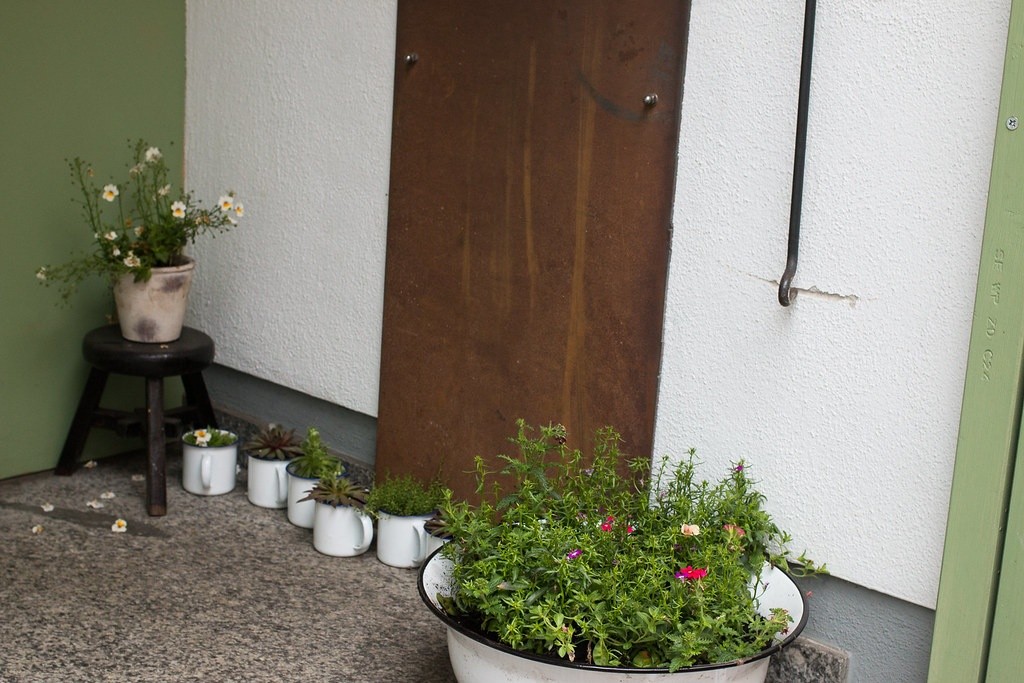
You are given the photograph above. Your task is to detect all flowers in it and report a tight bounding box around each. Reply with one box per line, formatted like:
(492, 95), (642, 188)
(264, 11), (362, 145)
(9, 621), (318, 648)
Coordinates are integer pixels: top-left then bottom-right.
(184, 426), (237, 446)
(35, 136), (245, 311)
(424, 420), (829, 666)
(33, 461), (145, 534)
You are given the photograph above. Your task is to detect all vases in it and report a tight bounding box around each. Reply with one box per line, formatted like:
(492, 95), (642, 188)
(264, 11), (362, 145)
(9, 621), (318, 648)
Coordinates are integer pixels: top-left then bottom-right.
(113, 254), (196, 342)
(182, 430), (238, 497)
(417, 519), (809, 683)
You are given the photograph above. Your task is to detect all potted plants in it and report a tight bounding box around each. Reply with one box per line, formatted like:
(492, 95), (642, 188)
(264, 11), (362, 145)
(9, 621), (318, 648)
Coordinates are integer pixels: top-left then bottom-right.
(285, 426), (350, 528)
(243, 424), (305, 509)
(296, 478), (374, 557)
(359, 470), (451, 569)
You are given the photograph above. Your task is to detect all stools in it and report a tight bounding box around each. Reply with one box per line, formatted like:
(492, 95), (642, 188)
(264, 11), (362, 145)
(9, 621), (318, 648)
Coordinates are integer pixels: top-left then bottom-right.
(55, 322), (215, 517)
